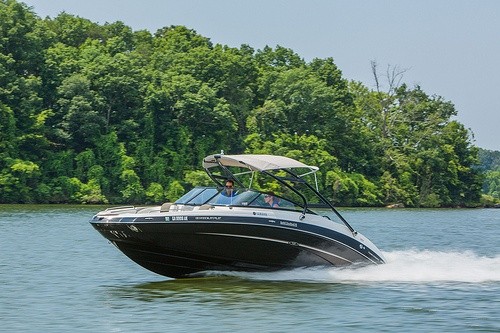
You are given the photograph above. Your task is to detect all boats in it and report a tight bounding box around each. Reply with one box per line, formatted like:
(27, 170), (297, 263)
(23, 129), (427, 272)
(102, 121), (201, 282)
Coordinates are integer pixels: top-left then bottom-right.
(89, 149), (387, 279)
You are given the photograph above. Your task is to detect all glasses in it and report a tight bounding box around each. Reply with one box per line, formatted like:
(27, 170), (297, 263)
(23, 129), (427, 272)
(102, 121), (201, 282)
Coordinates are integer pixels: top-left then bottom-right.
(225, 186), (233, 188)
(264, 196), (271, 198)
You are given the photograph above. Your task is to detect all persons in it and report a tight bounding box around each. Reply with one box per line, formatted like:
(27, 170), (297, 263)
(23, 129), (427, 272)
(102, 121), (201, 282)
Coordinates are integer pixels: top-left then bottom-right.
(213, 179), (241, 206)
(264, 191), (282, 209)
